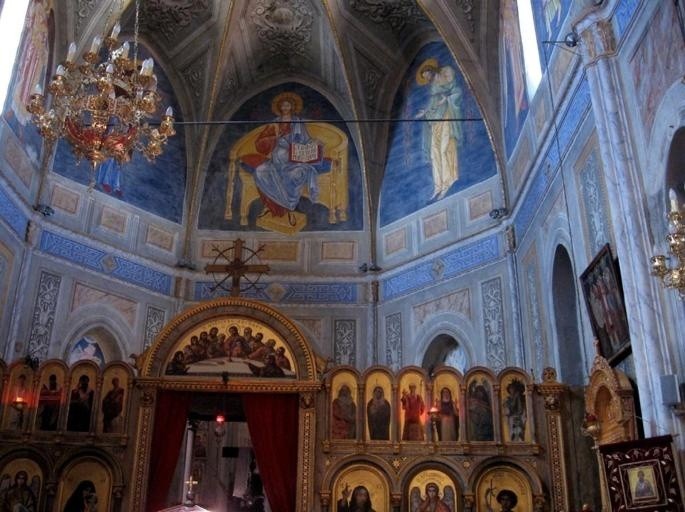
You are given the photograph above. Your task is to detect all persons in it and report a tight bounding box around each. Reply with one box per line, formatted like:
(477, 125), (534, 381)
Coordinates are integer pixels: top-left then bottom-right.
(5, 374), (29, 432)
(6, 0), (50, 141)
(66, 374), (93, 432)
(484, 484), (518, 512)
(502, 381), (528, 442)
(96, 117), (133, 199)
(434, 386), (459, 441)
(332, 383), (356, 438)
(415, 58), (465, 202)
(35, 374), (63, 433)
(61, 479), (98, 511)
(101, 376), (124, 433)
(336, 480), (375, 511)
(253, 91), (324, 227)
(468, 384), (494, 441)
(401, 382), (426, 441)
(0, 468), (37, 512)
(167, 324), (292, 378)
(202, 433), (208, 457)
(413, 480), (451, 511)
(634, 470), (653, 497)
(195, 434), (206, 457)
(582, 258), (632, 362)
(367, 384), (391, 440)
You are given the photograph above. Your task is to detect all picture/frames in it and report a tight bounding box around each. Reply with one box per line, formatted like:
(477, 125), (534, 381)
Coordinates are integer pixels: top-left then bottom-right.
(579, 243), (632, 367)
(595, 433), (685, 512)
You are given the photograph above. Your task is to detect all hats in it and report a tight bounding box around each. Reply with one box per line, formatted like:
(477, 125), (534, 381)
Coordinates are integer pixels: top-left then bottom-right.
(416, 58), (438, 86)
(272, 92), (303, 115)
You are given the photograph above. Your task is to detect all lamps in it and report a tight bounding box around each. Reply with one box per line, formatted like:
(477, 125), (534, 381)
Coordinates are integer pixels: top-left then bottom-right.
(26, 1), (177, 171)
(11, 397), (27, 430)
(214, 392), (226, 438)
(649, 188), (685, 309)
(427, 406), (442, 443)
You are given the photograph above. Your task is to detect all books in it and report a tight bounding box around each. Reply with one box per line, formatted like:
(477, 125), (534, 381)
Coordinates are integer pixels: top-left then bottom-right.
(288, 141), (322, 163)
